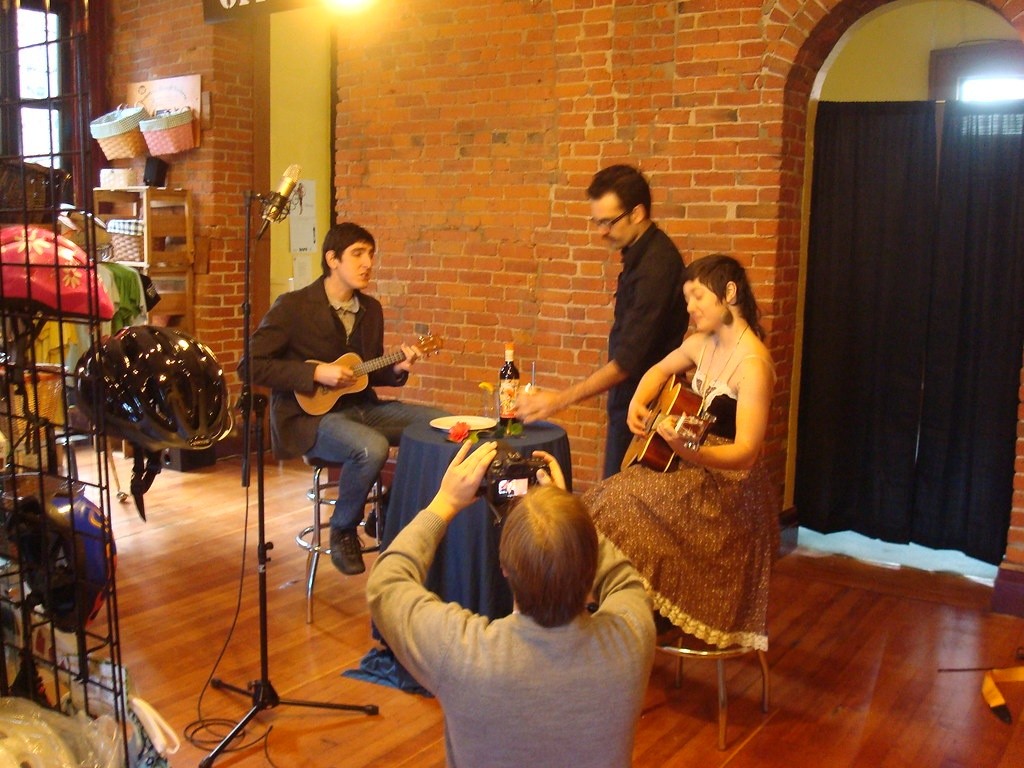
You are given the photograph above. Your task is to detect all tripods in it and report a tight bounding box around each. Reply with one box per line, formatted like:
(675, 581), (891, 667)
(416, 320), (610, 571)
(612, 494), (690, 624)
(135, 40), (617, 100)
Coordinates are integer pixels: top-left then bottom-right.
(199, 188), (376, 768)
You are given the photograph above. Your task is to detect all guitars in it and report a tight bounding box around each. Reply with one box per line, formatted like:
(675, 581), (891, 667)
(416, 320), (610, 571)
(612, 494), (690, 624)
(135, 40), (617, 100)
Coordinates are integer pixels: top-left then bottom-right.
(293, 330), (444, 416)
(619, 373), (717, 472)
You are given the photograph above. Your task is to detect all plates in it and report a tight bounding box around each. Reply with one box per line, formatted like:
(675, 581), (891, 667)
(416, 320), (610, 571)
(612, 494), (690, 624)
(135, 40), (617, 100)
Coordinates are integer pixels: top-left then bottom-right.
(429, 415), (498, 432)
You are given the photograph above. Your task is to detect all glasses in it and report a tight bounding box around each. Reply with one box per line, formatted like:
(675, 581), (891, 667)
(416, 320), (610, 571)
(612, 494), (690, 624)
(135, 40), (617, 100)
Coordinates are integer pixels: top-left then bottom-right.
(590, 203), (638, 234)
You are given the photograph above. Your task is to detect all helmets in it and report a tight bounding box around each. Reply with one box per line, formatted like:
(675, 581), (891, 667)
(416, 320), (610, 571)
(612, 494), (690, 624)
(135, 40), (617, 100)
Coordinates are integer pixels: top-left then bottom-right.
(0, 224), (115, 321)
(6, 493), (119, 634)
(73, 326), (234, 449)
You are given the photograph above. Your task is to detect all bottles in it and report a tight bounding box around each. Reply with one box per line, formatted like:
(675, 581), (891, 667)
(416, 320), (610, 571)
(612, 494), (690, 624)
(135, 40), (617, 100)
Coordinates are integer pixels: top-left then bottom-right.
(499, 350), (520, 426)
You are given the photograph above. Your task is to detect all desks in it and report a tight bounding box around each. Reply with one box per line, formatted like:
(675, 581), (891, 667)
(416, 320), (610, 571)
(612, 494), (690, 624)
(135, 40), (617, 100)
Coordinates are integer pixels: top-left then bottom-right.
(392, 420), (571, 617)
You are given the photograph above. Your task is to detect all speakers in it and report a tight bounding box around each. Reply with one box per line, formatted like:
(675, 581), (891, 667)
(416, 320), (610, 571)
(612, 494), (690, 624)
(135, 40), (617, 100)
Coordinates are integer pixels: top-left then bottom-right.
(143, 157), (168, 187)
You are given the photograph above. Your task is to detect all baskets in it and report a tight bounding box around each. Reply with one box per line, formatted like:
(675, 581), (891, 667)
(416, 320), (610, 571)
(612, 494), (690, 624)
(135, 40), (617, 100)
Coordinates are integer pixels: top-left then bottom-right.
(107, 220), (165, 262)
(0, 159), (71, 224)
(90, 107), (151, 161)
(139, 106), (195, 157)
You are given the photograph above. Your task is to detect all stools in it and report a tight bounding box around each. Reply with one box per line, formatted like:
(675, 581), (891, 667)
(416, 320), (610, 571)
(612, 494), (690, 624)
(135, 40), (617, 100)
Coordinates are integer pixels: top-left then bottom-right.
(295, 455), (383, 623)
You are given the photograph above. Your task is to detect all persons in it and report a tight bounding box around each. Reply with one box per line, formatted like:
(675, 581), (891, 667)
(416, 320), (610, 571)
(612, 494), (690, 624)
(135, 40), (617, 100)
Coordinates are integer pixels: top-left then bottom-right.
(235, 222), (452, 575)
(367, 441), (655, 767)
(516, 163), (690, 481)
(580, 254), (778, 651)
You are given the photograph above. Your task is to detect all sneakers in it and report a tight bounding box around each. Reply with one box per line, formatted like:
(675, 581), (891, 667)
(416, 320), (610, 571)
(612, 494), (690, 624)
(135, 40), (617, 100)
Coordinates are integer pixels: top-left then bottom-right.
(365, 513), (383, 540)
(329, 526), (365, 575)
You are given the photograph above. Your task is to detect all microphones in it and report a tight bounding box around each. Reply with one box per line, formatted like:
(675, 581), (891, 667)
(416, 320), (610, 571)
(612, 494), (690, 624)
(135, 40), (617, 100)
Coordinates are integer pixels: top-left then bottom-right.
(255, 164), (303, 241)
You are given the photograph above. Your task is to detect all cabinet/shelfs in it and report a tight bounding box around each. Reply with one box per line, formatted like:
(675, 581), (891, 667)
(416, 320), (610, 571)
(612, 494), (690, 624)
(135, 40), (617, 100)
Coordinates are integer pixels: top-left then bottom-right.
(91, 187), (196, 458)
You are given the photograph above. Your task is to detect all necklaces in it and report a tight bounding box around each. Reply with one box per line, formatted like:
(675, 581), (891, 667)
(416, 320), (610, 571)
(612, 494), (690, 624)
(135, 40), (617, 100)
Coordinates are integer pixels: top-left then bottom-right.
(700, 325), (749, 393)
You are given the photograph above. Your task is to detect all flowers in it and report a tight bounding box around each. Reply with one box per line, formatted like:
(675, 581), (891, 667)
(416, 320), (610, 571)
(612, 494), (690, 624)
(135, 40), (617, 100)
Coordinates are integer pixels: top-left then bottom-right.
(447, 422), (471, 443)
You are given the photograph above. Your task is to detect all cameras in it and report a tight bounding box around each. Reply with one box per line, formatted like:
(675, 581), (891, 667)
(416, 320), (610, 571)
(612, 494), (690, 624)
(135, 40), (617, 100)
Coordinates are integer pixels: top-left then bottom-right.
(476, 438), (550, 506)
